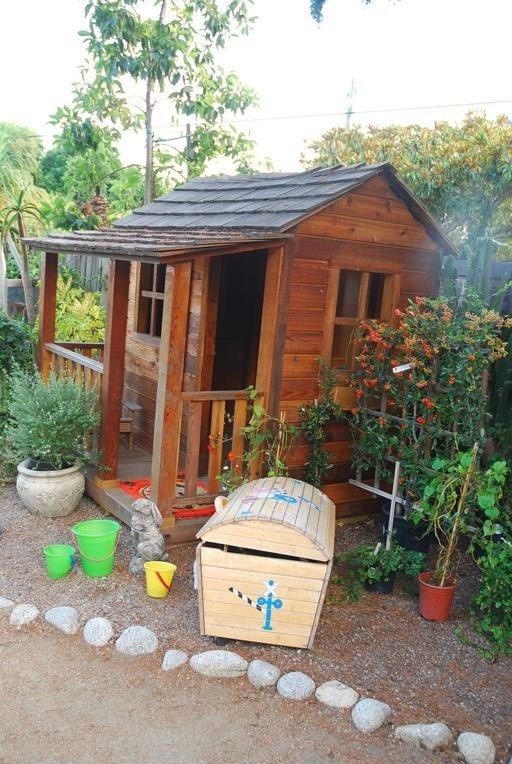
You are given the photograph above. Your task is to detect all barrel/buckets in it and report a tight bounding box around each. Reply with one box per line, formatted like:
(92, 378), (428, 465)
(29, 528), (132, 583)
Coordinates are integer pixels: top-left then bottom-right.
(418, 572), (458, 621)
(144, 561), (177, 598)
(44, 544), (74, 579)
(71, 520), (122, 576)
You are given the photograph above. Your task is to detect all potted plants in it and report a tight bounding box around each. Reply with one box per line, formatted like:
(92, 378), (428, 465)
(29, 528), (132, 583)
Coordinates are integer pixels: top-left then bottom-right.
(328, 434), (503, 623)
(2, 352), (112, 519)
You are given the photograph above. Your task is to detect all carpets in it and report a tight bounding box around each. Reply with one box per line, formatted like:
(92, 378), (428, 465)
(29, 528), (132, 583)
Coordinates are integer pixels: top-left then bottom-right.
(119, 471), (221, 518)
